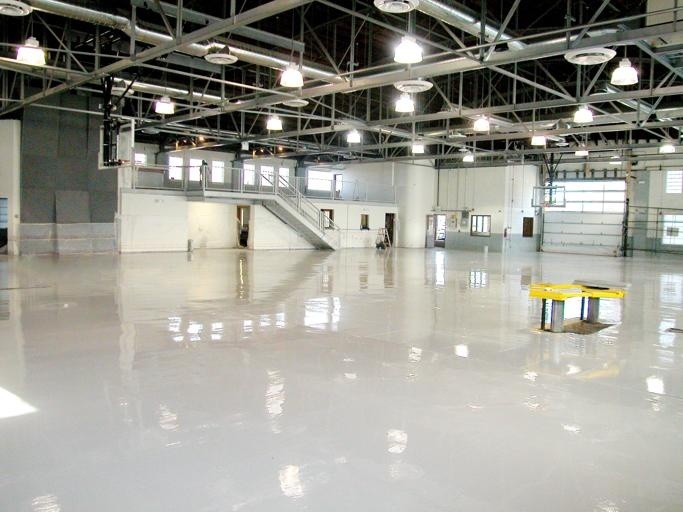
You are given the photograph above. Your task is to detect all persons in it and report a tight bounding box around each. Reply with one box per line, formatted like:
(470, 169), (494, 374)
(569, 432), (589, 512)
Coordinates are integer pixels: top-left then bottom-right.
(198, 159), (211, 190)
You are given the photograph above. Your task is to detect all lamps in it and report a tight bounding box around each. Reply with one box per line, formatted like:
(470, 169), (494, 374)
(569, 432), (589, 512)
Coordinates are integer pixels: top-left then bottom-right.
(574, 136), (589, 156)
(531, 109), (546, 146)
(16, 12), (46, 66)
(393, 12), (424, 64)
(412, 122), (423, 154)
(155, 59), (175, 114)
(462, 134), (475, 162)
(394, 92), (414, 113)
(346, 92), (361, 145)
(279, 9), (304, 88)
(572, 66), (594, 123)
(265, 105), (283, 130)
(659, 127), (675, 155)
(473, 69), (490, 131)
(610, 46), (639, 86)
